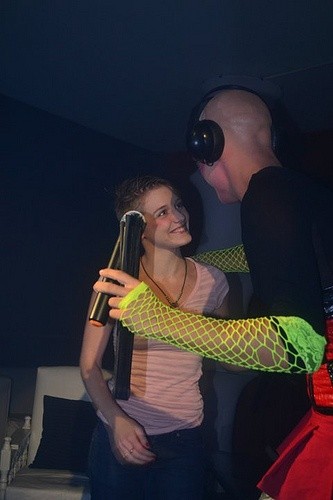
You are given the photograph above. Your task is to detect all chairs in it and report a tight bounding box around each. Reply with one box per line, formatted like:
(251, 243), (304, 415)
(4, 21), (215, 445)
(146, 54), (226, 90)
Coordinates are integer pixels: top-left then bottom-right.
(0, 367), (114, 500)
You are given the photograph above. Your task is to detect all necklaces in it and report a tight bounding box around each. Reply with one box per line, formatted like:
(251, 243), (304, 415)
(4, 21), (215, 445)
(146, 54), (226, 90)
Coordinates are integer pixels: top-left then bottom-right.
(139, 255), (188, 309)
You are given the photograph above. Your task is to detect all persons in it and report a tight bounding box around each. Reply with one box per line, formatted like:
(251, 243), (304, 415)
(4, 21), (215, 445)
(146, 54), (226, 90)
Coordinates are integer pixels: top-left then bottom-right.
(90, 88), (333, 500)
(78, 176), (249, 500)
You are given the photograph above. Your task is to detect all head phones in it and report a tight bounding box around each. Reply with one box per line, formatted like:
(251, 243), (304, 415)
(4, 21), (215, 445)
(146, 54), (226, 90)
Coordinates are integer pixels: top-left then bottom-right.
(185, 84), (283, 166)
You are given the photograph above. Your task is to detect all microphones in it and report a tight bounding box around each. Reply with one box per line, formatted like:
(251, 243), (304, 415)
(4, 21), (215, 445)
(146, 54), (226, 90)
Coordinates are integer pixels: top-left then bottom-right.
(88, 210), (146, 327)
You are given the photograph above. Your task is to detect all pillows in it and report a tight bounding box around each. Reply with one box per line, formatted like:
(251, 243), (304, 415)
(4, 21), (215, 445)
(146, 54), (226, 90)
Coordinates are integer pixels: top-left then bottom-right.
(29, 396), (97, 473)
